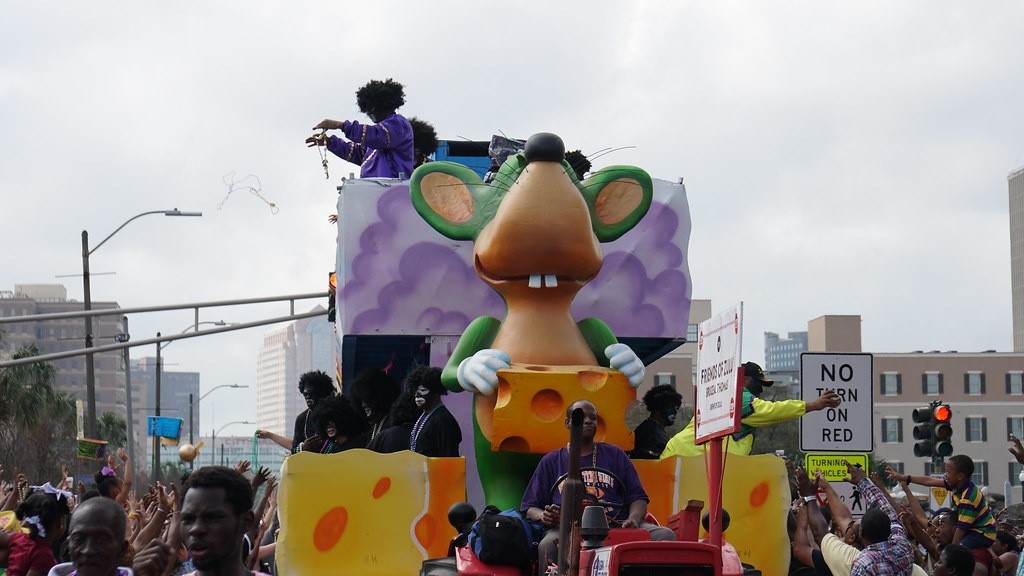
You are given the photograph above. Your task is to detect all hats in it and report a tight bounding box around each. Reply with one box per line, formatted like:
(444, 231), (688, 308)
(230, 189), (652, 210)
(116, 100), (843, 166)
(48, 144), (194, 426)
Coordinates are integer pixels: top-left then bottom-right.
(743, 362), (774, 387)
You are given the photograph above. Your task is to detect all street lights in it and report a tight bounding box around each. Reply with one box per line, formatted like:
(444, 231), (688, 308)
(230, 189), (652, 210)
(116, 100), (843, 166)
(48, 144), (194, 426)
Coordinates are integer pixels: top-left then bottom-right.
(1019, 470), (1024, 503)
(211, 420), (256, 467)
(153, 320), (242, 490)
(79, 207), (206, 473)
(187, 384), (250, 471)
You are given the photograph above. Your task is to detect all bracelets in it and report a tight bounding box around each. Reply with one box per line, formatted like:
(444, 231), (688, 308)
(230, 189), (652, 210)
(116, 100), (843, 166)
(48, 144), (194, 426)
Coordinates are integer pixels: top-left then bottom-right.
(297, 442), (304, 452)
(906, 475), (911, 486)
(803, 495), (817, 504)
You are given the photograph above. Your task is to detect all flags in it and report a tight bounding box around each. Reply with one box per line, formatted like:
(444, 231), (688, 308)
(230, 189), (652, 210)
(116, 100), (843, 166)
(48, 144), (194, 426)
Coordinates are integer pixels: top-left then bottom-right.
(77, 440), (108, 460)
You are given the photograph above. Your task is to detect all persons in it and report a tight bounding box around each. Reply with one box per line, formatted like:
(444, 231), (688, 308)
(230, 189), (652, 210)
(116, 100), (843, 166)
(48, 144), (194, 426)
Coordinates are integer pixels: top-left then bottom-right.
(291, 367), (463, 457)
(520, 400), (676, 576)
(787, 433), (1024, 576)
(0, 448), (280, 576)
(305, 79), (439, 224)
(255, 429), (292, 450)
(659, 362), (839, 460)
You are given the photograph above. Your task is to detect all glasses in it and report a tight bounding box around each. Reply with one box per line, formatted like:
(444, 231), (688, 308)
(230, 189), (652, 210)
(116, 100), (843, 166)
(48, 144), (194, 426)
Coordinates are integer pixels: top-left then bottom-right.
(848, 521), (856, 538)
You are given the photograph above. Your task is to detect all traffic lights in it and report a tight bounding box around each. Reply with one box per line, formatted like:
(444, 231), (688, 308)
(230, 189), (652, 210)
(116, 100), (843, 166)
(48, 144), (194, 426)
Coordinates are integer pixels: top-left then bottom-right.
(930, 405), (953, 457)
(327, 271), (336, 323)
(911, 406), (933, 458)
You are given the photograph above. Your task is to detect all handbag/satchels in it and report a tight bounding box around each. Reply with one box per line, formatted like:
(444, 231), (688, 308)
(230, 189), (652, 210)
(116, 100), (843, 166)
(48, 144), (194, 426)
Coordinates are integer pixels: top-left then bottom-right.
(467, 505), (534, 567)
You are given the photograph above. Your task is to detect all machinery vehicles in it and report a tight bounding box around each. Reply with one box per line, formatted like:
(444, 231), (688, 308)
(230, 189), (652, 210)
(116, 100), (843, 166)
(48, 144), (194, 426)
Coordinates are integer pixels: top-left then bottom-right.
(418, 407), (763, 576)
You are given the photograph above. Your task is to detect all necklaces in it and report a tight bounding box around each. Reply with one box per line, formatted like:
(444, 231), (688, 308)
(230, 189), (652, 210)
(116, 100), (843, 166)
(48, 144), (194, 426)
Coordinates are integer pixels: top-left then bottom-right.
(18, 481), (27, 502)
(314, 128), (329, 179)
(323, 442), (333, 454)
(372, 412), (389, 440)
(251, 430), (261, 475)
(422, 157), (427, 164)
(411, 400), (445, 452)
(567, 440), (596, 497)
(304, 410), (318, 439)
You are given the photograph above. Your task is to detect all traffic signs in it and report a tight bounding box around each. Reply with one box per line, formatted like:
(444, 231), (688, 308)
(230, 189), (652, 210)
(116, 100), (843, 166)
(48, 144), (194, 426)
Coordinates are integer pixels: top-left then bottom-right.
(804, 452), (869, 483)
